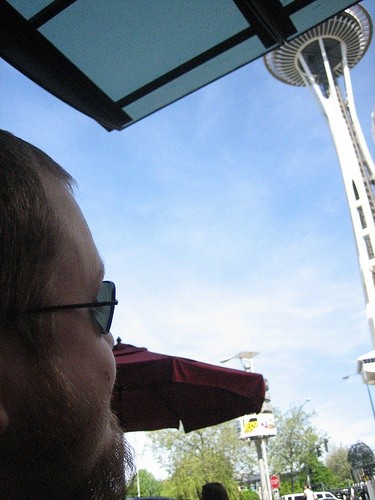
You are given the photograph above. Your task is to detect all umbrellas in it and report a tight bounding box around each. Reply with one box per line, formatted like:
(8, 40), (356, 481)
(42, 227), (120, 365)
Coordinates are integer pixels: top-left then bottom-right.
(111, 337), (265, 434)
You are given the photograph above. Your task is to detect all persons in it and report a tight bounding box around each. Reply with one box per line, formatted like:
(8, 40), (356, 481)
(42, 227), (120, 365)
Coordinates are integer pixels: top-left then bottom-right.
(200, 482), (229, 500)
(303, 484), (318, 500)
(1, 128), (136, 500)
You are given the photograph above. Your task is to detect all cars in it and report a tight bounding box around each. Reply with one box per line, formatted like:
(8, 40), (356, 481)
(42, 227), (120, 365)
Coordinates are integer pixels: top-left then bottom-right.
(281, 483), (369, 500)
